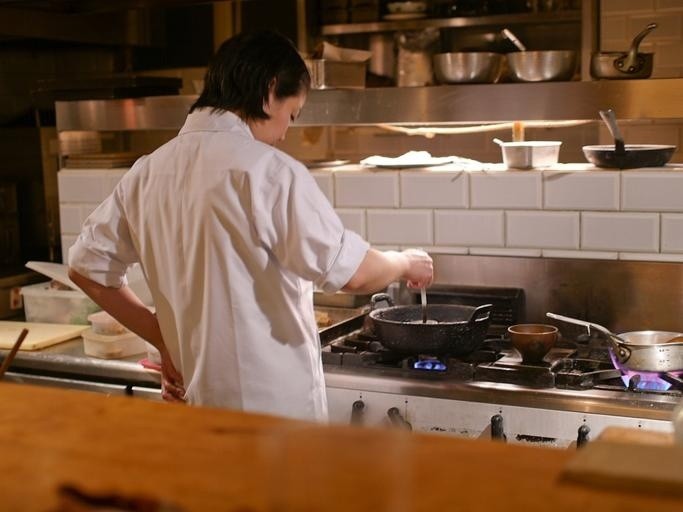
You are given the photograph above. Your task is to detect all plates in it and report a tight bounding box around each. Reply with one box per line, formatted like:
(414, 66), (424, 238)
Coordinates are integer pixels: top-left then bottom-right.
(360, 159), (454, 169)
(384, 11), (430, 21)
(307, 156), (349, 168)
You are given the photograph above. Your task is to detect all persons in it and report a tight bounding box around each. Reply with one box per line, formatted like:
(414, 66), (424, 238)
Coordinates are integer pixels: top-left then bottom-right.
(68, 28), (433, 426)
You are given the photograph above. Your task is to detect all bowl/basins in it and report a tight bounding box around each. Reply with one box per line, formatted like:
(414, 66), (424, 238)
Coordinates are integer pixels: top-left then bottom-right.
(430, 51), (506, 85)
(386, 1), (426, 12)
(506, 49), (579, 83)
(508, 323), (559, 364)
(500, 139), (564, 169)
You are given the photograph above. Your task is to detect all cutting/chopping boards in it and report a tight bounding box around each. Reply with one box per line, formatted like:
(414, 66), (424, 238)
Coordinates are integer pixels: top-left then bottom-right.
(0, 320), (92, 351)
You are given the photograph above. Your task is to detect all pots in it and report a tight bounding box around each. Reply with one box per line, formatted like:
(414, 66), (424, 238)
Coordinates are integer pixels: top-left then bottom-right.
(546, 312), (683, 373)
(368, 292), (494, 359)
(581, 108), (677, 170)
(589, 21), (658, 80)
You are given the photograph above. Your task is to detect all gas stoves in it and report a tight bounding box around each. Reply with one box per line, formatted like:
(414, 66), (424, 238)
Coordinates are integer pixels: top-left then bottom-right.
(315, 324), (683, 450)
